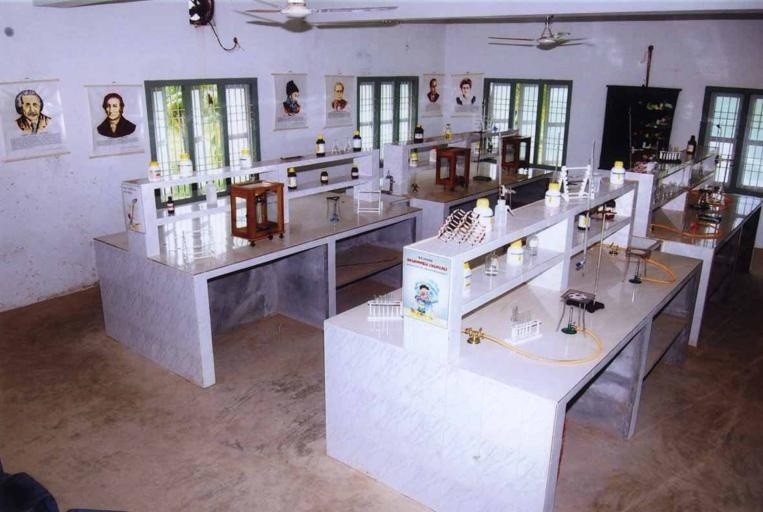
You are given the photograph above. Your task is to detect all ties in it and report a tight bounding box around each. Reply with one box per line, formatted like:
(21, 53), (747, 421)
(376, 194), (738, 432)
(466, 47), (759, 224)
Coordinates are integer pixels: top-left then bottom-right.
(32, 122), (37, 133)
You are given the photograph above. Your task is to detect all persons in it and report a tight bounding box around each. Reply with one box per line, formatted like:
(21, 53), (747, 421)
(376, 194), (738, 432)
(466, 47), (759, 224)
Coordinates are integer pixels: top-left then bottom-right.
(282, 80), (301, 117)
(425, 78), (440, 103)
(330, 82), (350, 113)
(11, 89), (53, 136)
(455, 78), (478, 106)
(95, 93), (135, 139)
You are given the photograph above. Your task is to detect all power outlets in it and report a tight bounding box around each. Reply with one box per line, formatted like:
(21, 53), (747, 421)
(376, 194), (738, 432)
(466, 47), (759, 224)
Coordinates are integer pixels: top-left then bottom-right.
(231, 33), (239, 45)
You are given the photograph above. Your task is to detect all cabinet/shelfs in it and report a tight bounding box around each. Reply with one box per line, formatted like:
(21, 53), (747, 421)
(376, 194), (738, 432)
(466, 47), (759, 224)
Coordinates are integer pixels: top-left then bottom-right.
(229, 180), (285, 245)
(403, 179), (639, 325)
(120, 147), (380, 237)
(614, 145), (720, 238)
(502, 136), (531, 172)
(384, 127), (517, 193)
(435, 147), (471, 191)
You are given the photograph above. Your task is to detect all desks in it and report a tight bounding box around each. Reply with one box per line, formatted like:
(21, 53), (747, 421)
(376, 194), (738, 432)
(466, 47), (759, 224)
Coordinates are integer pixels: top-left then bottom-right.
(324, 243), (704, 511)
(91, 192), (423, 389)
(631, 236), (662, 252)
(606, 190), (762, 348)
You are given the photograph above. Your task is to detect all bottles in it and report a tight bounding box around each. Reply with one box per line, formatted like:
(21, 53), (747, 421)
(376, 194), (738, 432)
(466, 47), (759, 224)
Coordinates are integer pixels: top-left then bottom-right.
(526, 235), (538, 257)
(686, 134), (696, 155)
(506, 240), (524, 269)
(288, 168), (297, 189)
(239, 147), (252, 167)
(413, 125), (424, 142)
(472, 197), (492, 236)
(350, 163), (359, 179)
(352, 130), (363, 152)
(442, 123), (452, 141)
(652, 181), (683, 206)
(370, 320), (390, 339)
(408, 149), (418, 169)
(544, 181), (561, 208)
(178, 153), (194, 178)
(494, 199), (508, 228)
(659, 151), (682, 163)
(315, 134), (325, 156)
(461, 263), (472, 299)
(509, 304), (535, 334)
(578, 212), (592, 231)
(320, 169), (328, 184)
(147, 160), (162, 182)
(429, 147), (437, 163)
(609, 161), (625, 187)
(370, 291), (397, 317)
(166, 196), (174, 214)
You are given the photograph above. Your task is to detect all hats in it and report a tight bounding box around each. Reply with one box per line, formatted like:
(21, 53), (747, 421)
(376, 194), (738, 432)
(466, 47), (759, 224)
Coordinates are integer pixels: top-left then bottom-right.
(286, 80), (299, 96)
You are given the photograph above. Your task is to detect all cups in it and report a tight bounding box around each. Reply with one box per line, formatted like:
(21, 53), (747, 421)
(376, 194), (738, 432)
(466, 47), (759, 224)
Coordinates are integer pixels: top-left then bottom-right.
(327, 196), (342, 225)
(485, 251), (497, 280)
(205, 184), (217, 208)
(588, 172), (602, 194)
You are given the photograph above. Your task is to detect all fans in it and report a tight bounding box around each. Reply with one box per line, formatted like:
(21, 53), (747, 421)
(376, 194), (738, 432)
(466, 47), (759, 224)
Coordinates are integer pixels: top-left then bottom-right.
(242, 0), (398, 19)
(488, 15), (588, 45)
